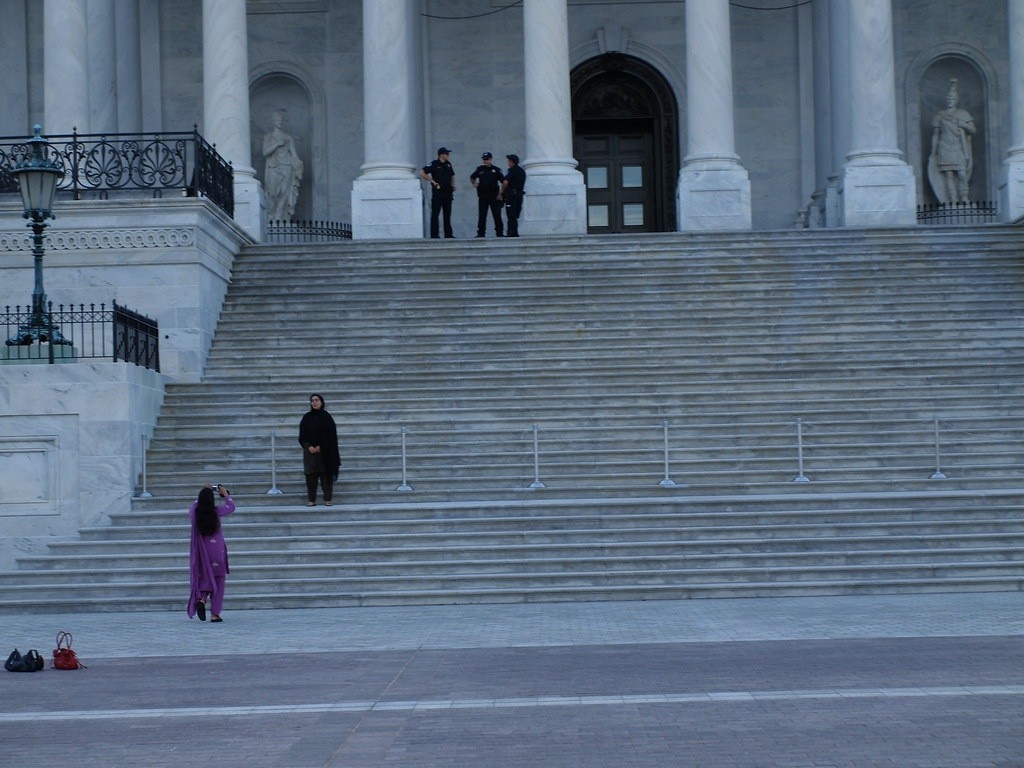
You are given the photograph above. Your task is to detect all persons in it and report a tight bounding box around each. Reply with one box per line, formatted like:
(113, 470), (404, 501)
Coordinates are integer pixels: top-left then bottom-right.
(261, 111), (303, 228)
(298, 394), (341, 507)
(187, 484), (236, 622)
(929, 89), (977, 205)
(420, 146), (456, 239)
(470, 151), (526, 238)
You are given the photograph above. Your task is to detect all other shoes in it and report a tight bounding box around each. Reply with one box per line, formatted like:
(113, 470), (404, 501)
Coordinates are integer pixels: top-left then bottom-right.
(307, 501), (315, 505)
(445, 235), (456, 238)
(325, 501), (332, 506)
(477, 235), (485, 237)
(497, 234), (504, 237)
(431, 235), (441, 238)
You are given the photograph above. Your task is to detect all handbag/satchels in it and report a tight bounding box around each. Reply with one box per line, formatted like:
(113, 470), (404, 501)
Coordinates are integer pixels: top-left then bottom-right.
(52, 631), (88, 670)
(5, 650), (44, 672)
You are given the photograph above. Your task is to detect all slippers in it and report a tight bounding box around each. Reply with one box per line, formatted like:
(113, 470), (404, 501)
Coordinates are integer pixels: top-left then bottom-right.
(197, 601), (207, 621)
(212, 616), (222, 622)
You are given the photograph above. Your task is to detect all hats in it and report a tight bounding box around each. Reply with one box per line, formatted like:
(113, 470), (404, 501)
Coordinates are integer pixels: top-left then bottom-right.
(506, 153), (519, 163)
(438, 147), (451, 153)
(482, 152), (492, 158)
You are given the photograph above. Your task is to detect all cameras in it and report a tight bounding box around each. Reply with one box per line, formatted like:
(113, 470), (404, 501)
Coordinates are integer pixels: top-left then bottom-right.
(211, 486), (218, 491)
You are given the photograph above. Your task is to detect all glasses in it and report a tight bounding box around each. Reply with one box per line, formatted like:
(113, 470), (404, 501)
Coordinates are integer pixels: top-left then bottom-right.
(444, 153), (449, 155)
(483, 158), (490, 161)
